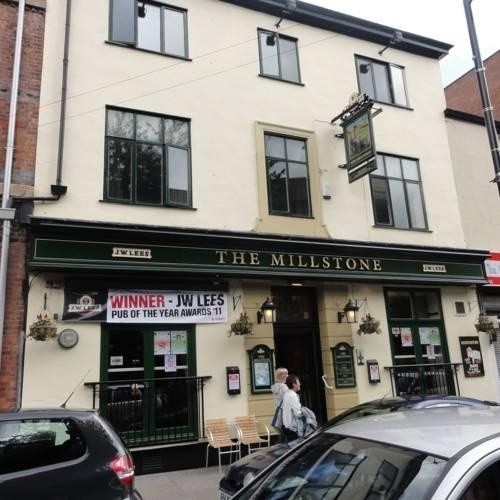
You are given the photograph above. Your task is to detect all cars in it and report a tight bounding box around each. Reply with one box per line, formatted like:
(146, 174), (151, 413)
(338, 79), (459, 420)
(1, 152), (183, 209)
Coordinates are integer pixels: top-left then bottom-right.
(220, 393), (500, 500)
(397, 371), (449, 394)
(0, 409), (136, 500)
(106, 383), (162, 418)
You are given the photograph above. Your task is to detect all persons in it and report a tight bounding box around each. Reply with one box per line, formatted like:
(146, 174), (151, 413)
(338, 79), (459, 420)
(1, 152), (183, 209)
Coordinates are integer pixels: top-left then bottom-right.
(281, 374), (304, 440)
(270, 368), (290, 442)
(351, 125), (362, 153)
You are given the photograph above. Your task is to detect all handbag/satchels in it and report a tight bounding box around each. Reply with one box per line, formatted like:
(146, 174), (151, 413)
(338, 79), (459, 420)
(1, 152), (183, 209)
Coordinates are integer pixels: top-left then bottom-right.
(271, 406), (284, 431)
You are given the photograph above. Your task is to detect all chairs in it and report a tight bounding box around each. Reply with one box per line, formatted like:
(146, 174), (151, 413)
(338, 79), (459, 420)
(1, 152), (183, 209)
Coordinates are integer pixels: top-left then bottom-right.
(205, 414), (271, 474)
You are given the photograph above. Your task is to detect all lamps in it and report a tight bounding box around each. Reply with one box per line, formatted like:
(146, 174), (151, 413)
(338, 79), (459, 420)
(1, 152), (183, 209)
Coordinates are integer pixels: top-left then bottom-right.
(338, 300), (359, 326)
(255, 297), (280, 326)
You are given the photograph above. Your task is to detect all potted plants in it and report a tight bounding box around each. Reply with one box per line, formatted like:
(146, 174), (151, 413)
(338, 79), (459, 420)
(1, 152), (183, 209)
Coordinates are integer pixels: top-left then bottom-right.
(229, 312), (255, 337)
(357, 314), (381, 336)
(30, 312), (58, 342)
(475, 312), (497, 340)
